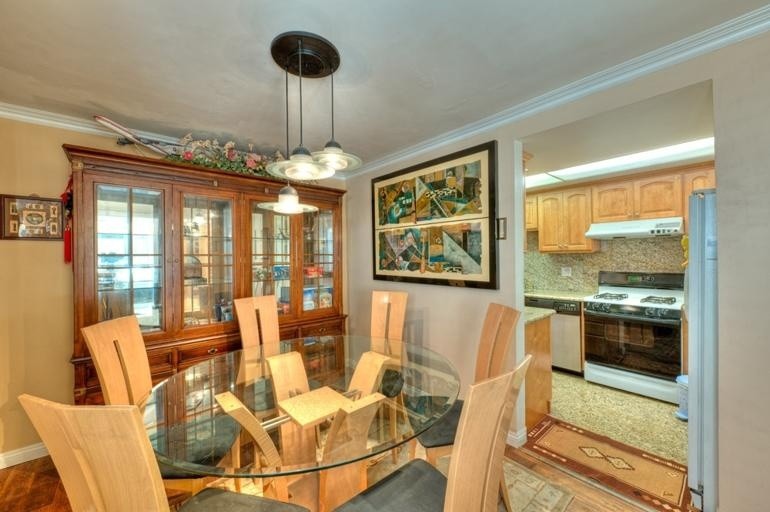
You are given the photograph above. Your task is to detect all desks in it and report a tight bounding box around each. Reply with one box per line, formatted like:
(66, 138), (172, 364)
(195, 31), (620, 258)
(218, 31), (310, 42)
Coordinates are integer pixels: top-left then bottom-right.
(524, 305), (556, 434)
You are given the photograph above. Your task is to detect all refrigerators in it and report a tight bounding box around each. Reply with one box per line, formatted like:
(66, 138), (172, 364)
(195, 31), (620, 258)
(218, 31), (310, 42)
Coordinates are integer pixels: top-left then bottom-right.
(686, 188), (719, 512)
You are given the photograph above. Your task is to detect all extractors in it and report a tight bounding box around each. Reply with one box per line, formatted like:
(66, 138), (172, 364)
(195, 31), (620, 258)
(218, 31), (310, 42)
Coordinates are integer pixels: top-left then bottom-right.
(585, 216), (685, 240)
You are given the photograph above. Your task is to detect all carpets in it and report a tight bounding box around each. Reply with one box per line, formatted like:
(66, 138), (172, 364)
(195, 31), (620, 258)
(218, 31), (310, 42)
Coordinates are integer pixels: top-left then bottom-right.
(523, 413), (691, 512)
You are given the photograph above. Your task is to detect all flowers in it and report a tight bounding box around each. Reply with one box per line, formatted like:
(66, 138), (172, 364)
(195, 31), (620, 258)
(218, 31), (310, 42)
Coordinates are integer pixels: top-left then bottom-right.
(165, 133), (284, 181)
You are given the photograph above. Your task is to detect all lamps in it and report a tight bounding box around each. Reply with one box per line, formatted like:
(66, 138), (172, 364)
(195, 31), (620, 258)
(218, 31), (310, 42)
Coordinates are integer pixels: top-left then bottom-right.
(254, 30), (363, 217)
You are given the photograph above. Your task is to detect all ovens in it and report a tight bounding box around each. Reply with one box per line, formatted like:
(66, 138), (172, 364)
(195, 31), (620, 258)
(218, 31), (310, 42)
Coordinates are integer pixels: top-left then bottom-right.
(584, 310), (682, 405)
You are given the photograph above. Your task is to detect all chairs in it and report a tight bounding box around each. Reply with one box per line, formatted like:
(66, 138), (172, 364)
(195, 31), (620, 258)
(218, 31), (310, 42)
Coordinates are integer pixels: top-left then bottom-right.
(19, 392), (313, 511)
(370, 289), (415, 465)
(332, 353), (533, 512)
(233, 295), (280, 487)
(403, 303), (522, 511)
(80, 315), (244, 494)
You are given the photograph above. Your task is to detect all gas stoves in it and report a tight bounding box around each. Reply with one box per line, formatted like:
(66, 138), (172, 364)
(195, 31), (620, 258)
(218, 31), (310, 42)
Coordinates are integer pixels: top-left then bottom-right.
(584, 292), (684, 311)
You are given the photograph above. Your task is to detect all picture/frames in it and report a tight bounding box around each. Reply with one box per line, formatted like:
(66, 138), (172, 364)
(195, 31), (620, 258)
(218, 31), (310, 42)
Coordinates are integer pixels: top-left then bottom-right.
(371, 140), (499, 290)
(0, 194), (64, 241)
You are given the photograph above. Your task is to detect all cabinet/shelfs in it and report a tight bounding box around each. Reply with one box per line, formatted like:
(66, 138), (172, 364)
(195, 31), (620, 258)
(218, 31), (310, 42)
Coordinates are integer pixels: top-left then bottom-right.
(592, 171), (682, 222)
(683, 162), (715, 234)
(525, 194), (538, 230)
(62, 142), (349, 406)
(537, 186), (600, 254)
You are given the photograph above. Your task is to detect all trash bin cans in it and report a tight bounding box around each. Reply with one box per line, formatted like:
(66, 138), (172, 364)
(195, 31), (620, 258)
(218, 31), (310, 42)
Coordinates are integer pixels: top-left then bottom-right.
(674, 375), (689, 421)
